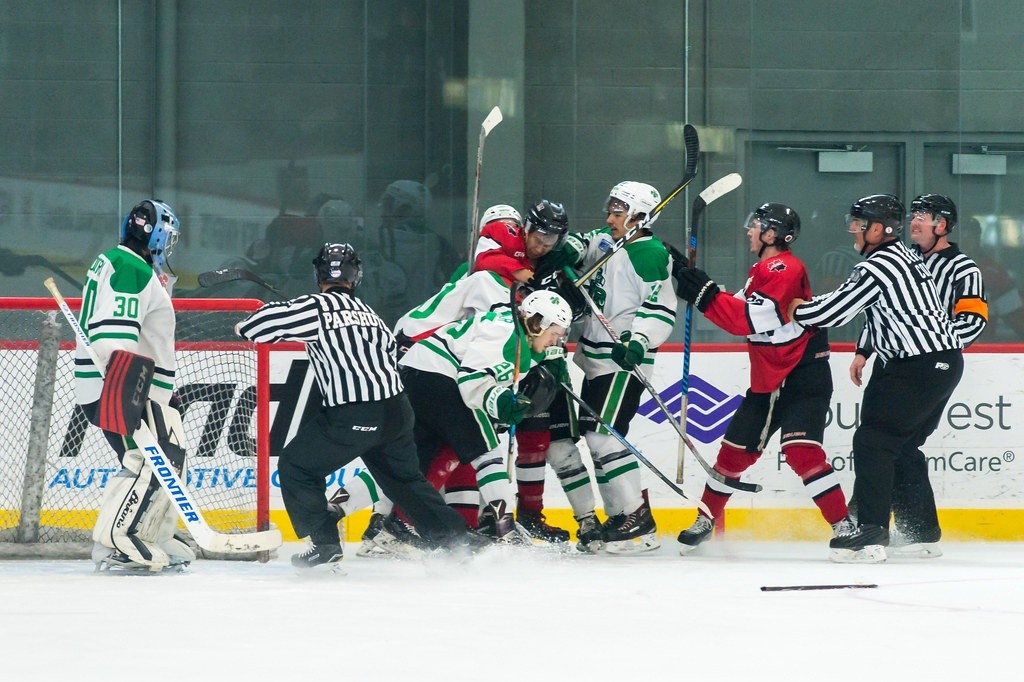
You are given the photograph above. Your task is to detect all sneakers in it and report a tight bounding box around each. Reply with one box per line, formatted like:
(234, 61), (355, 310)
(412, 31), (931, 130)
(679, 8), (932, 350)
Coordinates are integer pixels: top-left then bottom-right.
(368, 489), (660, 553)
(830, 516), (857, 535)
(677, 514), (716, 556)
(829, 524), (890, 564)
(889, 515), (942, 558)
(291, 537), (348, 581)
(326, 486), (351, 553)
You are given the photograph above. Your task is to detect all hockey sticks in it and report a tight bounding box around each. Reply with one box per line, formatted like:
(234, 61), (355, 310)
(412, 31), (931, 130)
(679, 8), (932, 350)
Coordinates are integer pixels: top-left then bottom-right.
(676, 171), (743, 484)
(560, 382), (714, 520)
(467, 106), (503, 277)
(44, 276), (283, 554)
(507, 337), (520, 478)
(573, 124), (700, 288)
(564, 264), (763, 492)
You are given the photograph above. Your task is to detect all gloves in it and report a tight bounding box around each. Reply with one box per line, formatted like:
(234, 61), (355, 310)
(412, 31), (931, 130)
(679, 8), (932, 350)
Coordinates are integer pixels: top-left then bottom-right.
(611, 332), (649, 372)
(394, 328), (415, 361)
(662, 240), (689, 277)
(675, 266), (721, 314)
(552, 232), (587, 270)
(525, 269), (567, 296)
(483, 385), (533, 425)
(543, 345), (572, 390)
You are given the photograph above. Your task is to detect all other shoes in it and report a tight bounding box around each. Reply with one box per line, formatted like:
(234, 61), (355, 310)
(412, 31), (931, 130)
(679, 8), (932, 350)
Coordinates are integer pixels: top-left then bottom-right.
(91, 542), (148, 569)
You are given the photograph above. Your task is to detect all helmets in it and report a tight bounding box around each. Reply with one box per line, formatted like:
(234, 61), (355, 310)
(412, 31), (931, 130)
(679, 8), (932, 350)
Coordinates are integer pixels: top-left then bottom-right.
(524, 198), (569, 249)
(517, 290), (572, 336)
(314, 202), (367, 248)
(610, 181), (661, 229)
(479, 204), (523, 231)
(120, 199), (181, 275)
(850, 194), (906, 237)
(312, 241), (363, 291)
(911, 193), (958, 233)
(375, 178), (434, 232)
(756, 201), (801, 245)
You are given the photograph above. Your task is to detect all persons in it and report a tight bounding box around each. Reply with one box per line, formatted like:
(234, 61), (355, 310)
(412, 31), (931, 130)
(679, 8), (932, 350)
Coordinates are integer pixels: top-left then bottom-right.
(953, 216), (1024, 345)
(233, 242), (489, 568)
(357, 181), (677, 560)
(814, 248), (865, 343)
(661, 203), (856, 553)
(847, 193), (989, 541)
(71, 199), (196, 576)
(213, 181), (450, 346)
(787, 193), (964, 566)
